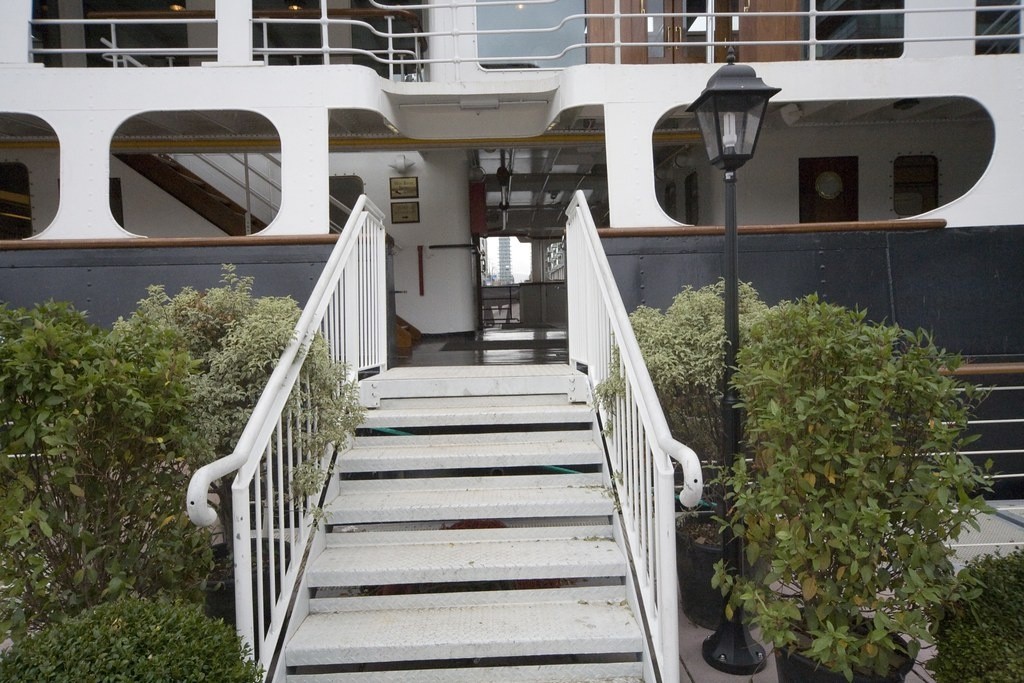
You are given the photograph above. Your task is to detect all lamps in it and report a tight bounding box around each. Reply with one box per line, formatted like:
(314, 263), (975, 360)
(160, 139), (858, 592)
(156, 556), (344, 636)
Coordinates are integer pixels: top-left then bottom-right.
(386, 154), (415, 172)
(768, 103), (803, 131)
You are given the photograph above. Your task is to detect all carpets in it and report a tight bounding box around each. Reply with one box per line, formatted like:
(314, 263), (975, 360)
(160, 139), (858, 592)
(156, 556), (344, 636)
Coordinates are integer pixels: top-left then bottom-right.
(440, 340), (566, 351)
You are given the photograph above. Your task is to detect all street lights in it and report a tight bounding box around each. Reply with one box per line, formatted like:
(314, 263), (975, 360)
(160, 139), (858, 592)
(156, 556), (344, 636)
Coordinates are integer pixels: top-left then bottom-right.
(685, 46), (782, 676)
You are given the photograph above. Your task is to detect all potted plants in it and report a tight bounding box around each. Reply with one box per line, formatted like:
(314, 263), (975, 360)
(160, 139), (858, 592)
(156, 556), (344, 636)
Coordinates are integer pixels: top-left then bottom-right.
(590, 276), (1003, 682)
(111, 263), (368, 662)
